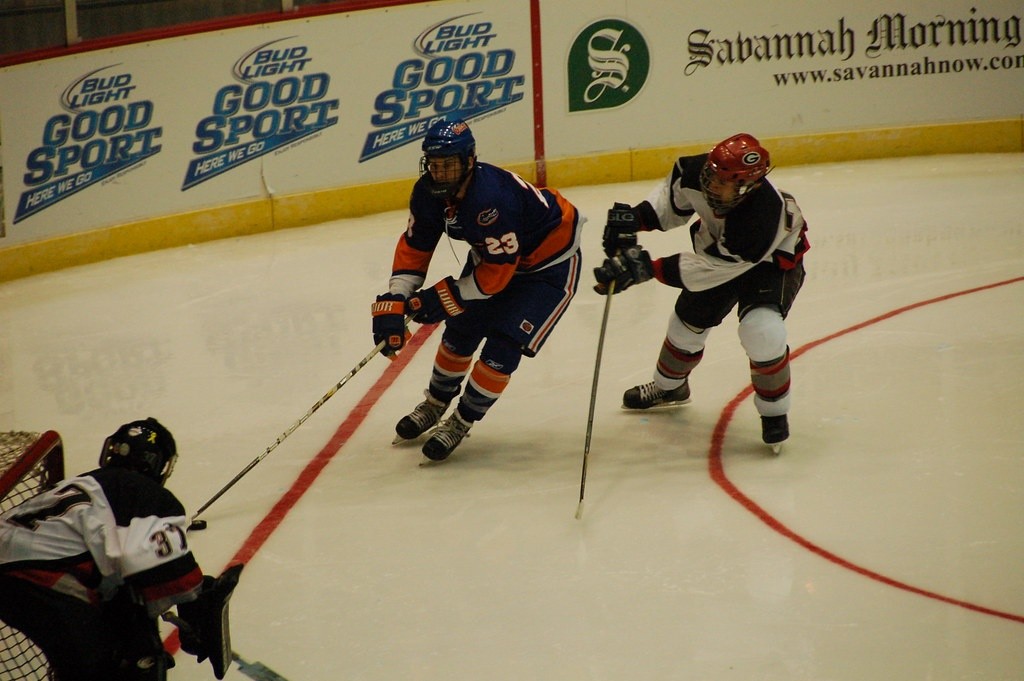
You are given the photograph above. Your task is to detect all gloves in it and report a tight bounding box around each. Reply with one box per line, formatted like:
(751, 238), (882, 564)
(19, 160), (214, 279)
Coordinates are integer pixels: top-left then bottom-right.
(602, 202), (655, 259)
(593, 245), (656, 295)
(404, 276), (468, 324)
(373, 293), (412, 361)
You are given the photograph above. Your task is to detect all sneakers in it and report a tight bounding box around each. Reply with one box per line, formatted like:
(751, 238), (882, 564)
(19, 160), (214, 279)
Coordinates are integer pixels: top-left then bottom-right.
(419, 413), (471, 467)
(391, 398), (448, 445)
(760, 415), (790, 455)
(621, 376), (692, 414)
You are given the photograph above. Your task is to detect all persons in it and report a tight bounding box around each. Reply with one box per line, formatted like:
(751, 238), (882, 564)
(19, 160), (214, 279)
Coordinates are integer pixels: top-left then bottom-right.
(593, 133), (811, 453)
(371, 119), (581, 465)
(0, 417), (236, 681)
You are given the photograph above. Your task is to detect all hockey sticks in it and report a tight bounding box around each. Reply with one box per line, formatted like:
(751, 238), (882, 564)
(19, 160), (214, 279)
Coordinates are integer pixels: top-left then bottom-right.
(161, 611), (290, 681)
(185, 288), (419, 531)
(574, 255), (623, 521)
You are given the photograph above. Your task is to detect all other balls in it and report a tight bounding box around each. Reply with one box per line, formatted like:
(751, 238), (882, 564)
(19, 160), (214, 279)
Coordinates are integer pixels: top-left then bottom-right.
(187, 519), (206, 530)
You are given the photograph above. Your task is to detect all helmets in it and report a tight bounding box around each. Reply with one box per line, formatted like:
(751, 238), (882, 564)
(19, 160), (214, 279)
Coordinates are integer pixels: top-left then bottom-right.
(419, 118), (476, 198)
(99, 417), (178, 487)
(699, 133), (770, 208)
